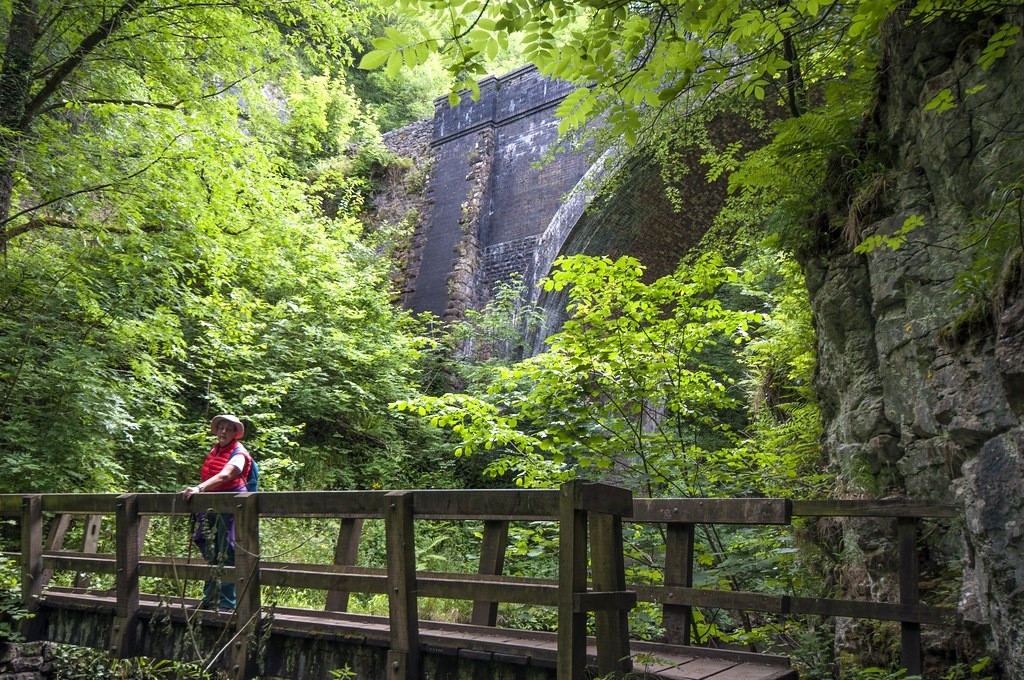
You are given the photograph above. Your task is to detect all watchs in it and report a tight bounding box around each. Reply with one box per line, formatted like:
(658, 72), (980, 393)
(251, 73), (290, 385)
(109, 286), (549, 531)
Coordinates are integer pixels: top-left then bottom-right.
(197, 484), (204, 492)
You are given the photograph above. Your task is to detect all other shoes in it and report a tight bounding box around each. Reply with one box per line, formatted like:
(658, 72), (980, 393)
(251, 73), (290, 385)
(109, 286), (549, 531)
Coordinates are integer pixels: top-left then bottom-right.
(218, 608), (235, 613)
(186, 604), (217, 610)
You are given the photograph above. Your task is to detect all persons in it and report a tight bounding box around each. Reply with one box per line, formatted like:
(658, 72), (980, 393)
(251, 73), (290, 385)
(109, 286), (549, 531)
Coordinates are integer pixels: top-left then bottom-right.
(178, 415), (251, 613)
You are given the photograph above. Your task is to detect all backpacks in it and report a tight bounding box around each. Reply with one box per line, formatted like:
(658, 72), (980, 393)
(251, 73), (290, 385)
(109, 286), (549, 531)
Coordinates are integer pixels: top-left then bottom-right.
(229, 447), (258, 492)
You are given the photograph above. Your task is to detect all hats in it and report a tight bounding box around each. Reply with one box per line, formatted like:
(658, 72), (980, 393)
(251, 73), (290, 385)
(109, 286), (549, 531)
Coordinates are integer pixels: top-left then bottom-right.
(211, 415), (244, 439)
